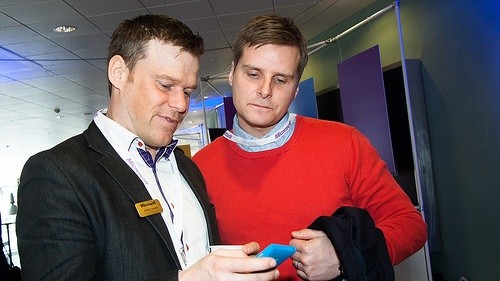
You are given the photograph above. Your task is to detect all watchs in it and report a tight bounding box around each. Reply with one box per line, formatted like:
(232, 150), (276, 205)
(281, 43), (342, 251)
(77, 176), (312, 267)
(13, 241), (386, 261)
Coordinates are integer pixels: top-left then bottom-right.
(339, 261), (347, 275)
(190, 14), (428, 281)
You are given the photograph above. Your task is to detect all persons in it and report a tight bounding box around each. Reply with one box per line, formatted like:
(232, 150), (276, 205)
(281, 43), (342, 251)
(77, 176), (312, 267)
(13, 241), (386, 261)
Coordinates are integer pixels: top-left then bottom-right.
(15, 15), (280, 281)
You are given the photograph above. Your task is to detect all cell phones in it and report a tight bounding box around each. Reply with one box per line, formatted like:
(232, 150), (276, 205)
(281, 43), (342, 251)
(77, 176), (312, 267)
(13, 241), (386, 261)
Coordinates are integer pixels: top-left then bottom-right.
(256, 243), (297, 273)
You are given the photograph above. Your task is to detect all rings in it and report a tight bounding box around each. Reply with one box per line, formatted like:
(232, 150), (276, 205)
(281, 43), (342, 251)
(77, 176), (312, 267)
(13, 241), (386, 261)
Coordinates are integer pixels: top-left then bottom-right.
(296, 261), (300, 268)
